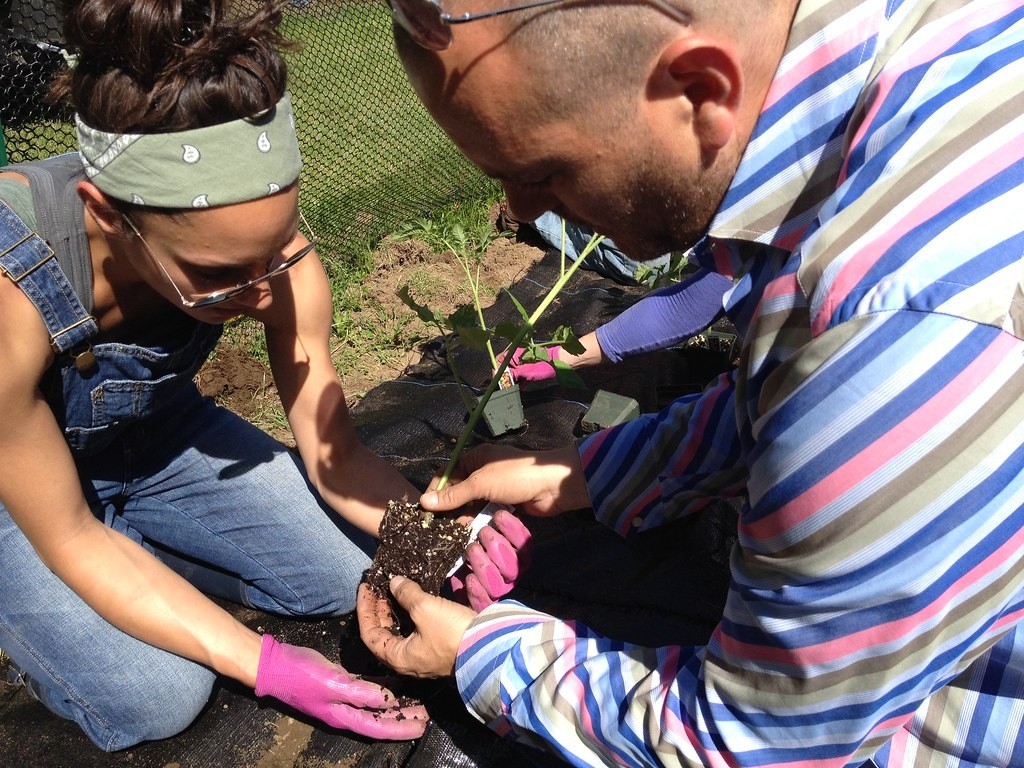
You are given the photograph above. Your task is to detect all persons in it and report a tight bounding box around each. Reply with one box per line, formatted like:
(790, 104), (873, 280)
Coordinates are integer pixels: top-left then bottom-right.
(0, 0), (539, 753)
(496, 199), (741, 383)
(358, 2), (1023, 768)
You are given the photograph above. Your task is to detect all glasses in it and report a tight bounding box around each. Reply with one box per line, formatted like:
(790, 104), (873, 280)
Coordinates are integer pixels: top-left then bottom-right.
(380, 0), (572, 50)
(111, 207), (320, 307)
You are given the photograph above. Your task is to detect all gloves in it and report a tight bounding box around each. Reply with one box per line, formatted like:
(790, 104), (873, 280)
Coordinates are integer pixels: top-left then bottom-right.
(491, 345), (563, 382)
(447, 509), (532, 611)
(255, 632), (428, 739)
